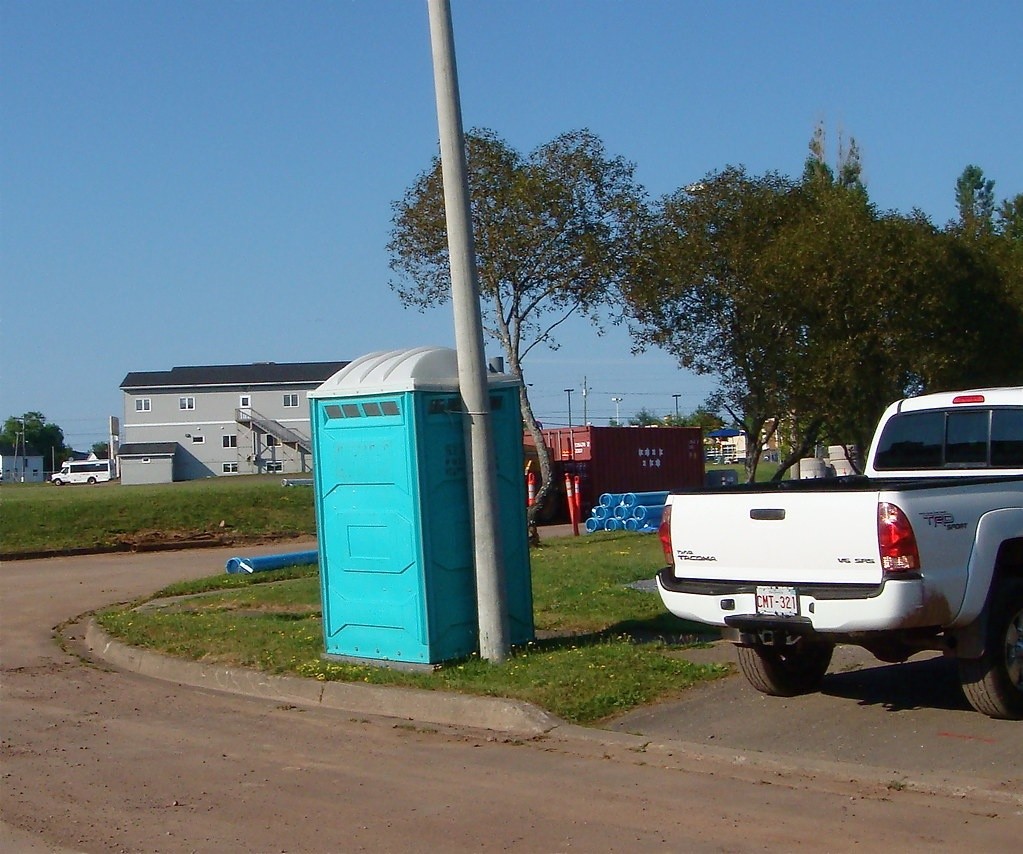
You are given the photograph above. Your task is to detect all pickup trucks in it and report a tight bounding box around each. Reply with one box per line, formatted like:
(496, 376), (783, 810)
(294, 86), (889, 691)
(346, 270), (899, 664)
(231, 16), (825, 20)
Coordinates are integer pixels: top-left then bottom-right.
(657, 385), (1023, 721)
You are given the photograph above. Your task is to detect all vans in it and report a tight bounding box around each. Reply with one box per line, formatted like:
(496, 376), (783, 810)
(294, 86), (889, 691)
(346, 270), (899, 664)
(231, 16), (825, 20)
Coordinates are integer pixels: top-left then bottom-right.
(51, 459), (115, 486)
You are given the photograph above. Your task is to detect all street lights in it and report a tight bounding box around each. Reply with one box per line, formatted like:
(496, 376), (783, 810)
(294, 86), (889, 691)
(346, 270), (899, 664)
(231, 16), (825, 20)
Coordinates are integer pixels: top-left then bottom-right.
(612, 397), (623, 425)
(564, 389), (574, 428)
(672, 394), (681, 419)
(18, 419), (26, 483)
